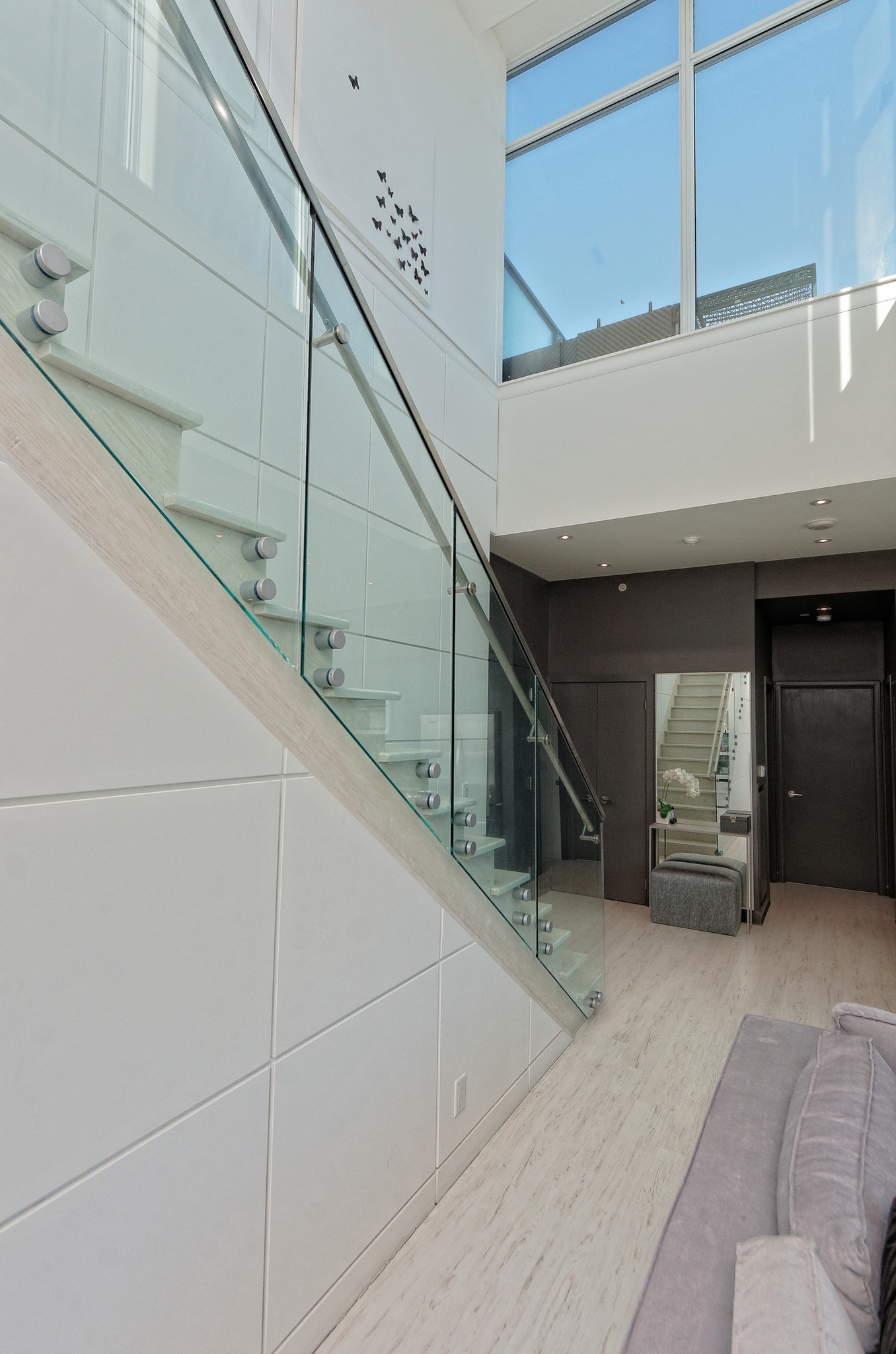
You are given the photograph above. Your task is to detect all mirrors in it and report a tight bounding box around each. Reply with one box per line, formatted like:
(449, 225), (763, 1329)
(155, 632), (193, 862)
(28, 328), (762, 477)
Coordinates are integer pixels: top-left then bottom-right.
(655, 672), (754, 910)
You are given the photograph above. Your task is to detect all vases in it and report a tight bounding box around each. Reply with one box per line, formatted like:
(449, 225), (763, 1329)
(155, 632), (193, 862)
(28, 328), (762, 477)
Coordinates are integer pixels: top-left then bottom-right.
(656, 810), (674, 824)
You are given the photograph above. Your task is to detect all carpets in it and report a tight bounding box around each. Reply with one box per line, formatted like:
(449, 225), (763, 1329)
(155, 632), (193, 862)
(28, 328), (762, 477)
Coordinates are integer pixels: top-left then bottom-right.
(620, 1012), (829, 1354)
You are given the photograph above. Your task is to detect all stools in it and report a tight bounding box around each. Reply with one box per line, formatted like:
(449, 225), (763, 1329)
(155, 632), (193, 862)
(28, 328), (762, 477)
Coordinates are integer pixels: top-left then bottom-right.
(650, 861), (747, 937)
(662, 852), (747, 908)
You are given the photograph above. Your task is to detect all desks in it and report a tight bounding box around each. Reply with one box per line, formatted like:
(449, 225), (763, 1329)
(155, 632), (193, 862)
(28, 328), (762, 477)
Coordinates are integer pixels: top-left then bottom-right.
(649, 819), (752, 935)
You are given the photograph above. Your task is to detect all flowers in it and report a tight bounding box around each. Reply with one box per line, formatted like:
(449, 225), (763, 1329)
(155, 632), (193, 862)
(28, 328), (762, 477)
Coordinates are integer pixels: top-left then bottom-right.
(659, 767), (696, 818)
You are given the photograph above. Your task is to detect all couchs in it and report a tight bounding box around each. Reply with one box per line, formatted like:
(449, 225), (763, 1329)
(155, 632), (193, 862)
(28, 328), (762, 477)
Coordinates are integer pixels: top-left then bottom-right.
(734, 1002), (896, 1354)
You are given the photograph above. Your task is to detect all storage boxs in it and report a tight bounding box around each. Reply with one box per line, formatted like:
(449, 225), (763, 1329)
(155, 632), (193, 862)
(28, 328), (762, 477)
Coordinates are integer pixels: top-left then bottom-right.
(720, 813), (751, 835)
